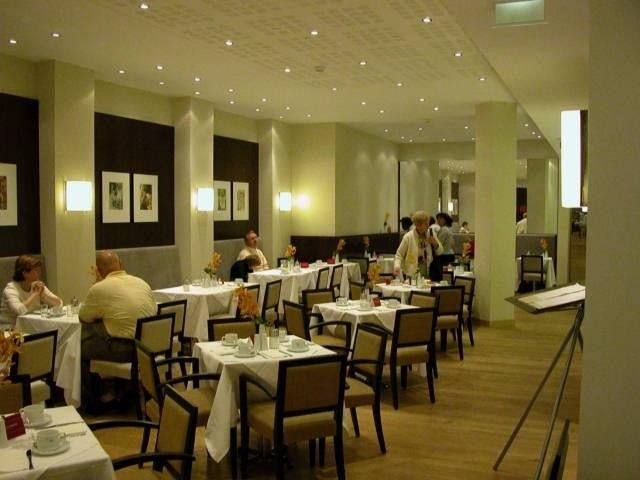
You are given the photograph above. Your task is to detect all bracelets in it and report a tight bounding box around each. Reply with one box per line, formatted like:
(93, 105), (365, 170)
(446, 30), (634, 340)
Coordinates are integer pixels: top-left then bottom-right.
(434, 244), (439, 250)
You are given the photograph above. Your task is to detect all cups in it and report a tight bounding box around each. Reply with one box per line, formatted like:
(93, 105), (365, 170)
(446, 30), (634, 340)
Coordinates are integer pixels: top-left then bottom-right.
(17, 404), (46, 423)
(223, 329), (306, 354)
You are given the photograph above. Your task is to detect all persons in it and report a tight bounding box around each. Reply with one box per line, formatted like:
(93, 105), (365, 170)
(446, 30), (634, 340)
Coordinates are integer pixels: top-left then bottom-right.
(459, 221), (470, 233)
(437, 212), (455, 280)
(516, 212), (527, 234)
(399, 216), (416, 244)
(78, 250), (158, 418)
(237, 230), (270, 272)
(230, 254), (261, 283)
(0, 253), (63, 331)
(429, 216), (440, 236)
(393, 210), (444, 282)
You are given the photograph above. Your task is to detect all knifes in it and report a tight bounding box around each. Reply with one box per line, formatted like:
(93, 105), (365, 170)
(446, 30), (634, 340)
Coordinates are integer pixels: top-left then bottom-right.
(26, 448), (34, 469)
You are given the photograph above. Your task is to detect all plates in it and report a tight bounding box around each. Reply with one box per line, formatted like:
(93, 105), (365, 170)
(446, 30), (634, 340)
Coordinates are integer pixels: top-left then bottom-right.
(21, 414), (69, 456)
(220, 338), (310, 358)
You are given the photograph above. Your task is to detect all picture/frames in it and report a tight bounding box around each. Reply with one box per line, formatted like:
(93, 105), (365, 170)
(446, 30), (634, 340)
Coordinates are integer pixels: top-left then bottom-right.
(212, 180), (251, 224)
(0, 162), (20, 229)
(99, 170), (161, 225)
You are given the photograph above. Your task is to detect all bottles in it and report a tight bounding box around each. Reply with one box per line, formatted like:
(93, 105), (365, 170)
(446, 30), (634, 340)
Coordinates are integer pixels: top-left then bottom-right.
(384, 273), (449, 288)
(183, 275), (243, 290)
(336, 296), (400, 310)
(35, 295), (80, 318)
(279, 257), (322, 274)
(526, 250), (531, 256)
(447, 256), (469, 274)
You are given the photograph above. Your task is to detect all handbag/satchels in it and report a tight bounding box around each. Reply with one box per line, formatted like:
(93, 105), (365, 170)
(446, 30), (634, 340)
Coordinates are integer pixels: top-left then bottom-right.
(429, 258), (444, 281)
